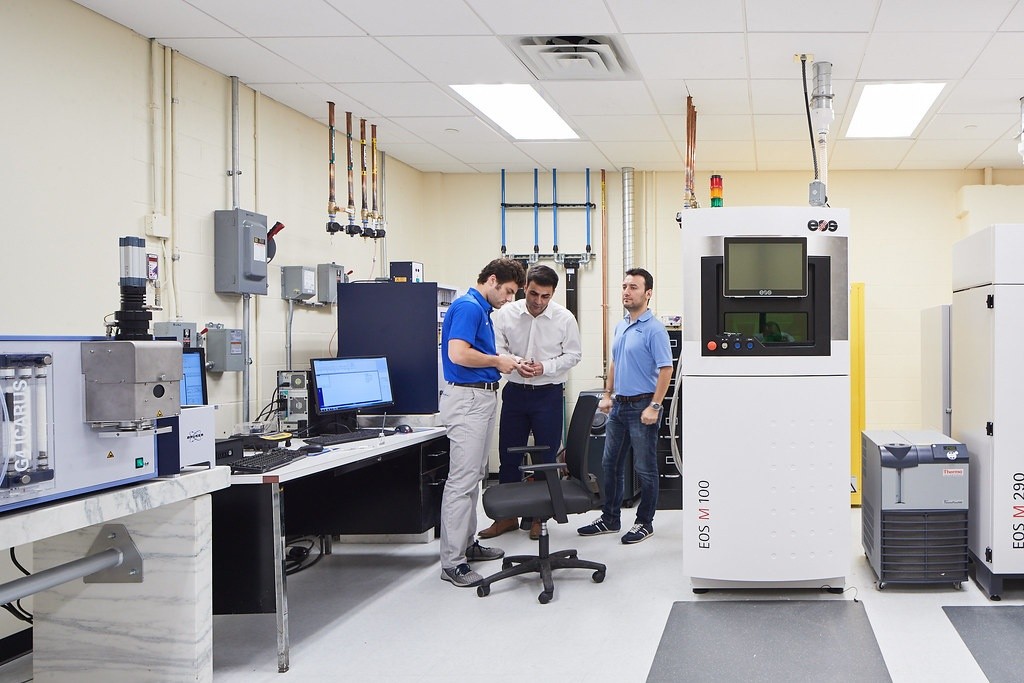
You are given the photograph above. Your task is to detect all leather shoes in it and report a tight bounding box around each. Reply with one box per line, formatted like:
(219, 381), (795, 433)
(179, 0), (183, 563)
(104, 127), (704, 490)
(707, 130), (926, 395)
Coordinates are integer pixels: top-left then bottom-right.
(530, 517), (541, 539)
(478, 515), (519, 537)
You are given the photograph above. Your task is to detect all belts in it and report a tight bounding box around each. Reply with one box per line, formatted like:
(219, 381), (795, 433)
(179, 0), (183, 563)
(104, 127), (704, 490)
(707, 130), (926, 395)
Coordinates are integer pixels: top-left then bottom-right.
(616, 394), (654, 403)
(448, 381), (499, 390)
(508, 381), (563, 391)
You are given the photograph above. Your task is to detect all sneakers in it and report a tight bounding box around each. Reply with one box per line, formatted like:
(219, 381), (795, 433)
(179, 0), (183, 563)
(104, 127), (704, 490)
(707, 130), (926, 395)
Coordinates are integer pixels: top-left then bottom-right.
(621, 522), (654, 543)
(441, 564), (483, 586)
(466, 540), (505, 560)
(577, 518), (620, 536)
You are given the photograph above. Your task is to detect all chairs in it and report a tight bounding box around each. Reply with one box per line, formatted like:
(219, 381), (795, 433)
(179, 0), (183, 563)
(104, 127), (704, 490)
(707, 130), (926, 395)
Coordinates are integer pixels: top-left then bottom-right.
(477, 394), (607, 604)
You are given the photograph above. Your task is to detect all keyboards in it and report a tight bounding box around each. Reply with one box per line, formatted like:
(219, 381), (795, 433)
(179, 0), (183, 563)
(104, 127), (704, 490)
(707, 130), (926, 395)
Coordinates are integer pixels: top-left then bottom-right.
(227, 448), (306, 475)
(303, 430), (396, 446)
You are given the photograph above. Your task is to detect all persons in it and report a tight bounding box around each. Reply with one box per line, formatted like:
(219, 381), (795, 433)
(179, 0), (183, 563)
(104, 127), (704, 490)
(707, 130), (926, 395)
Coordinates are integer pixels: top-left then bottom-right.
(477, 265), (582, 538)
(439, 258), (520, 586)
(577, 267), (674, 543)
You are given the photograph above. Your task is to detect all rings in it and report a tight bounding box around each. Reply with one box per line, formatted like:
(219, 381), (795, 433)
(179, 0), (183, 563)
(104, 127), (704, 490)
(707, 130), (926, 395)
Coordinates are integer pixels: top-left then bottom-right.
(533, 371), (535, 374)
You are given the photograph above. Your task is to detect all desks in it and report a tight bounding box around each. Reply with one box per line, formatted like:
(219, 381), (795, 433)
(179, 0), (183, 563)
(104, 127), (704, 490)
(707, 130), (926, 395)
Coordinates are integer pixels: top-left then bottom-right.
(0, 466), (231, 683)
(213, 426), (448, 674)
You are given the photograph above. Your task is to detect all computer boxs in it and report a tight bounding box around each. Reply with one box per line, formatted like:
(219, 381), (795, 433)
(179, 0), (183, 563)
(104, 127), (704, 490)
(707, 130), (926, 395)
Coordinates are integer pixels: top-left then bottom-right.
(277, 370), (356, 439)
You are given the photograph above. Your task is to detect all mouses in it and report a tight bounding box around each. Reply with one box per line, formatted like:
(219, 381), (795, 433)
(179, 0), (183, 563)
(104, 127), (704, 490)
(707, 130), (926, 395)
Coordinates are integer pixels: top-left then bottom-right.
(298, 444), (323, 453)
(395, 425), (413, 433)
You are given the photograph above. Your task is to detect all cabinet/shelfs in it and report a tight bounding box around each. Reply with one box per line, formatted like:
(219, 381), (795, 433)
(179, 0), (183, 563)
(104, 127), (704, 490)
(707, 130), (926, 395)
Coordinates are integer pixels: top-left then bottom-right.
(656, 329), (682, 510)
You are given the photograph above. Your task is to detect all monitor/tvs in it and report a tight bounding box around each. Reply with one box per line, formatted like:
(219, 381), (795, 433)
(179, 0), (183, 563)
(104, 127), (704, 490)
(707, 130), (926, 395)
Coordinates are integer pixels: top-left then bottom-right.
(181, 348), (209, 406)
(310, 356), (395, 434)
(724, 237), (809, 298)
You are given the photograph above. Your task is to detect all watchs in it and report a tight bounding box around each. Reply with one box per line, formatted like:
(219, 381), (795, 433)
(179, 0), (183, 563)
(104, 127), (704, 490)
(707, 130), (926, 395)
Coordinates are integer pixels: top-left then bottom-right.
(649, 402), (662, 411)
